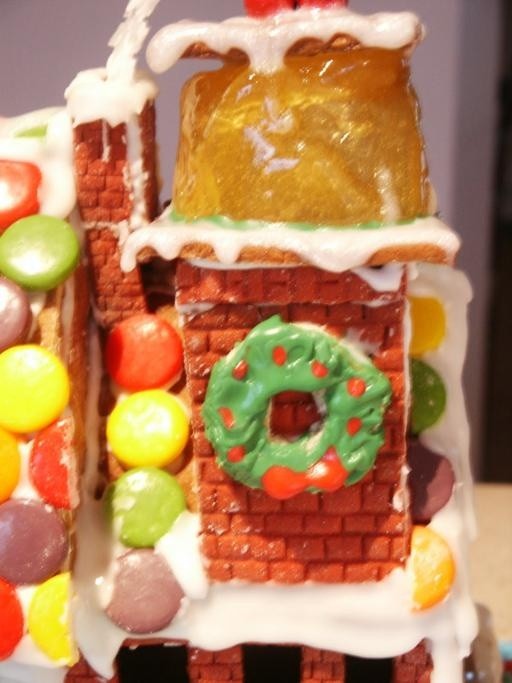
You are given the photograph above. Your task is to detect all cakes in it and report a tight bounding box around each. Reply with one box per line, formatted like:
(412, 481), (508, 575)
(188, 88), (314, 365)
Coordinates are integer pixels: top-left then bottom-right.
(1, 0), (501, 683)
